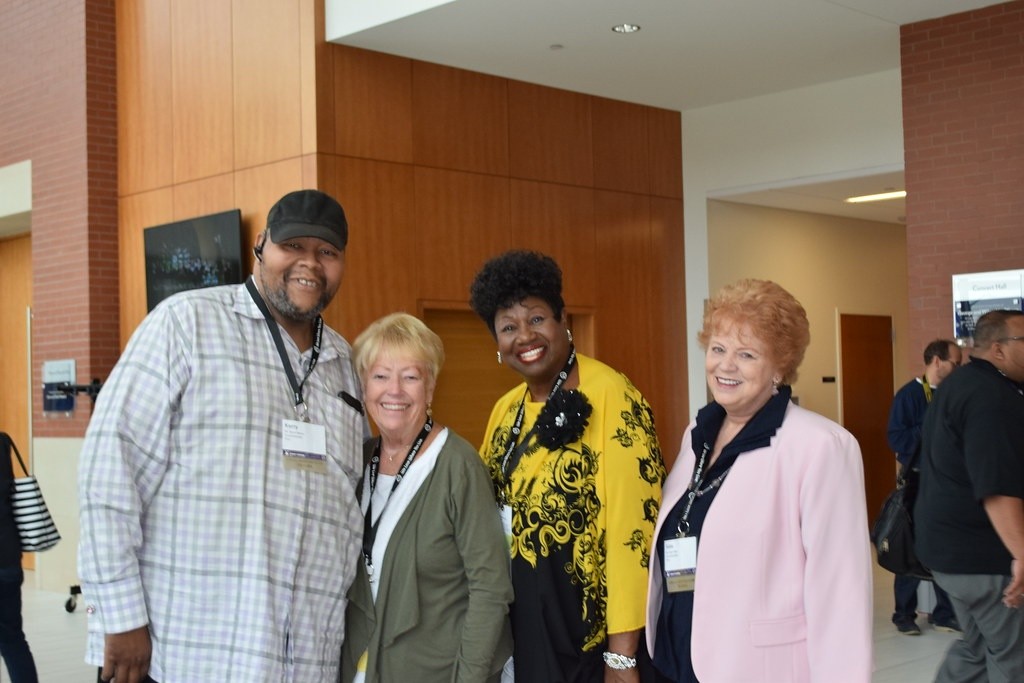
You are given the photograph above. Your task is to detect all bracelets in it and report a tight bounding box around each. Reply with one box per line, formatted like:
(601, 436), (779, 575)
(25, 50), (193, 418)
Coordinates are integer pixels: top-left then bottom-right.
(603, 651), (636, 672)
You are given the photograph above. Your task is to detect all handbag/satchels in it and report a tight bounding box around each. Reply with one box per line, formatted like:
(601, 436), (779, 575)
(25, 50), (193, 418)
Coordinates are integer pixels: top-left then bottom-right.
(1, 432), (61, 552)
(870, 486), (933, 581)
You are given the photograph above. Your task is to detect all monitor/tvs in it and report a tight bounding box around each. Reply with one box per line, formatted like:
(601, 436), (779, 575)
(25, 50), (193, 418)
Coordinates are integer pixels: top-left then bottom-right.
(144, 209), (243, 315)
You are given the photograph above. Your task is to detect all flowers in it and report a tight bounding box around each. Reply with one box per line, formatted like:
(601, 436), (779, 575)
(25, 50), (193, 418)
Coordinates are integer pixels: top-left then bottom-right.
(535, 386), (593, 446)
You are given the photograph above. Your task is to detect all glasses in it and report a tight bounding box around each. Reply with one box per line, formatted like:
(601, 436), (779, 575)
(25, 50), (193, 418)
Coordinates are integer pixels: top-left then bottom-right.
(942, 357), (961, 369)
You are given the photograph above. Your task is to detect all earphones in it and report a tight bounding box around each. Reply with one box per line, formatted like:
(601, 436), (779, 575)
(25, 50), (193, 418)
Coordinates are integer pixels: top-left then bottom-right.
(254, 246), (263, 263)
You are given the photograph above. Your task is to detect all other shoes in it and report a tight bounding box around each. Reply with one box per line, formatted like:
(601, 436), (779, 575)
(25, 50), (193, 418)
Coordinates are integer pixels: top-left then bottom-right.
(898, 622), (920, 635)
(933, 617), (962, 633)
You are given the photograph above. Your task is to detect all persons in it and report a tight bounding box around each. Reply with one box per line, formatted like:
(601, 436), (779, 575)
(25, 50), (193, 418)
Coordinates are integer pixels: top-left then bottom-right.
(469, 247), (669, 683)
(914, 308), (1024, 683)
(0, 431), (63, 683)
(645, 277), (874, 683)
(888, 338), (964, 637)
(336, 312), (511, 682)
(76, 188), (366, 682)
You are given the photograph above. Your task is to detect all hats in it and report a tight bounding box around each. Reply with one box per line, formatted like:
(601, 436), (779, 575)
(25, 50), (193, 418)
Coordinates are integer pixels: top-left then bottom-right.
(266, 190), (348, 252)
